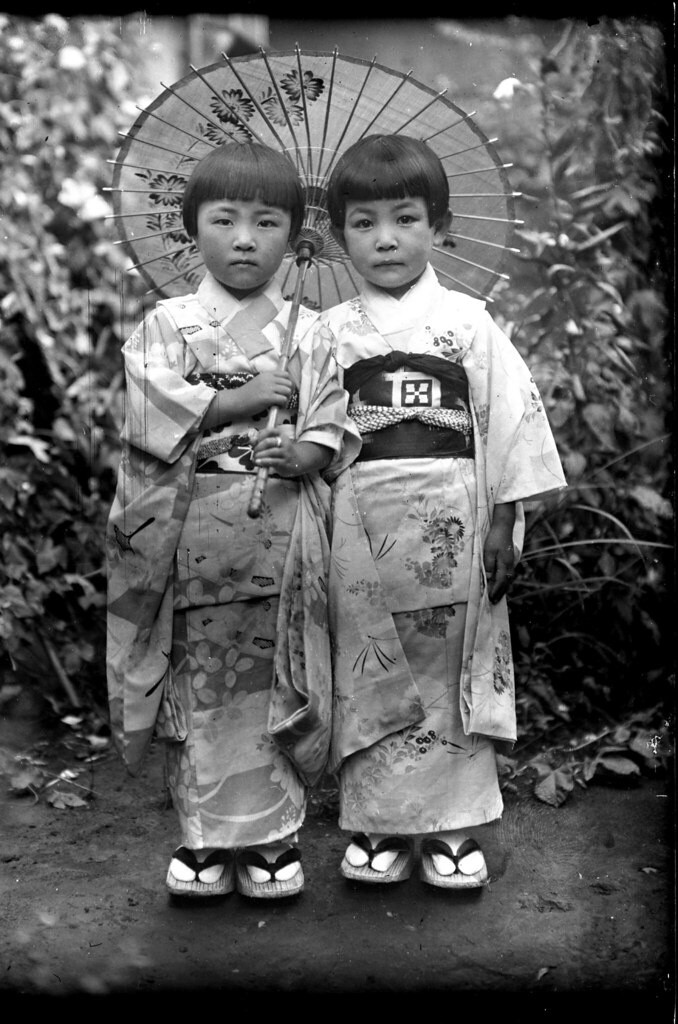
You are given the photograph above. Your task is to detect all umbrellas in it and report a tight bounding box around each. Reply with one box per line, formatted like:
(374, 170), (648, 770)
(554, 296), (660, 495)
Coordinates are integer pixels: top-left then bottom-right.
(99, 41), (525, 520)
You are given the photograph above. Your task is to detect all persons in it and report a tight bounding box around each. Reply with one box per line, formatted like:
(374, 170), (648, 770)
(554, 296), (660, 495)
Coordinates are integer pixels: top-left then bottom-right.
(322, 137), (568, 890)
(106, 142), (363, 898)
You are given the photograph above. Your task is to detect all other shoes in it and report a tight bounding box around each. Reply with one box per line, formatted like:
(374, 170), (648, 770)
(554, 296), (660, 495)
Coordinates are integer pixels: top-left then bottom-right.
(420, 839), (488, 889)
(340, 832), (414, 883)
(236, 849), (305, 898)
(165, 844), (234, 896)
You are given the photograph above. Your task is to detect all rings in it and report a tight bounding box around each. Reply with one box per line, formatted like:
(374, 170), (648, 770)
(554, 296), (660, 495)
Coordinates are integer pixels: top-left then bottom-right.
(507, 574), (513, 577)
(277, 436), (281, 447)
